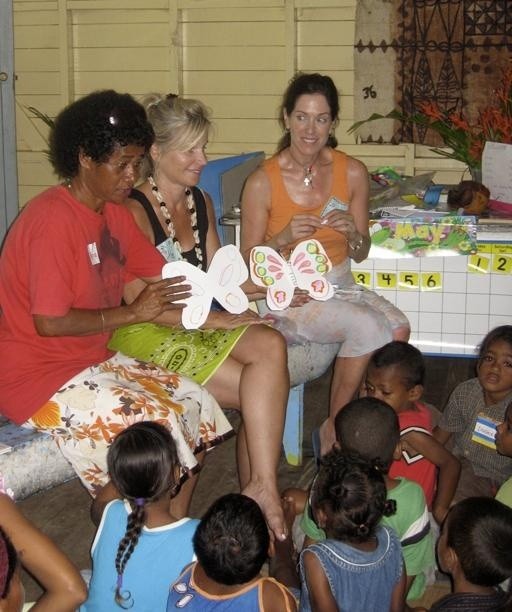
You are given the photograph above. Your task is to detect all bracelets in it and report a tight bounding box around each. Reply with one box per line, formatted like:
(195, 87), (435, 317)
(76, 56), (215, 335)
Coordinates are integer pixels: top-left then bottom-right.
(347, 236), (365, 252)
(95, 308), (108, 337)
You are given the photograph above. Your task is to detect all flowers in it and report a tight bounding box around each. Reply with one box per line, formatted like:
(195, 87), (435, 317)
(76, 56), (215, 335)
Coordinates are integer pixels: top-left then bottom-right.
(344, 94), (512, 187)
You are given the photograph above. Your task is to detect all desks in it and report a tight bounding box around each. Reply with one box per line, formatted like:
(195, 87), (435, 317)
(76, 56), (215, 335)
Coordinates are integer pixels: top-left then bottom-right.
(351, 188), (512, 358)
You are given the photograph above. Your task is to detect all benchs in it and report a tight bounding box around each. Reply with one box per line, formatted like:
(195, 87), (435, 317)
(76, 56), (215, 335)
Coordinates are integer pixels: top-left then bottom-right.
(0, 331), (343, 503)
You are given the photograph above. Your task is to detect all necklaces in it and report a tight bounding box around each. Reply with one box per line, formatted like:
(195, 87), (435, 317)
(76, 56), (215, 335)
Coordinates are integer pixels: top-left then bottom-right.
(145, 170), (202, 273)
(287, 149), (320, 185)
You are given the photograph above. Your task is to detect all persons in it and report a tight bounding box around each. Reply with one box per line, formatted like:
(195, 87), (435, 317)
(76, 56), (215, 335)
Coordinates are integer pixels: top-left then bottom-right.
(75, 421), (202, 612)
(1, 88), (236, 526)
(238, 71), (413, 460)
(122, 91), (292, 561)
(284, 325), (512, 612)
(165, 493), (301, 612)
(0, 493), (88, 612)
